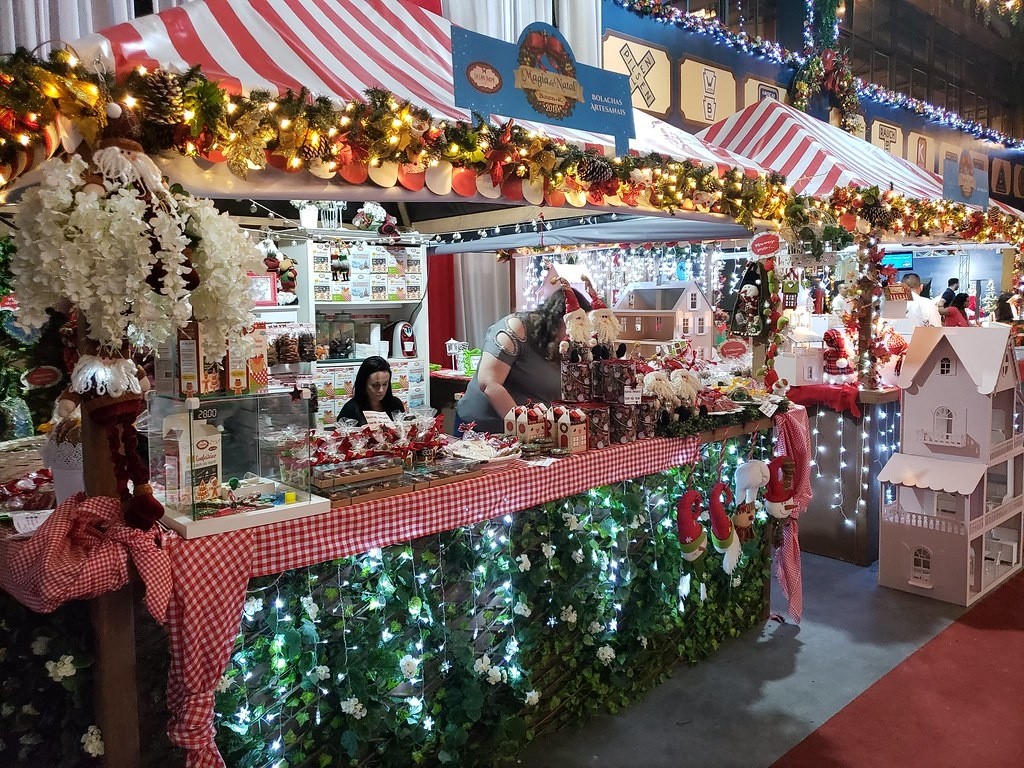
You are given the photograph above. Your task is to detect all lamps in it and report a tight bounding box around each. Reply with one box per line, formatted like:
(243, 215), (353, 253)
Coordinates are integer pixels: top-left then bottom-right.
(446, 338), (459, 373)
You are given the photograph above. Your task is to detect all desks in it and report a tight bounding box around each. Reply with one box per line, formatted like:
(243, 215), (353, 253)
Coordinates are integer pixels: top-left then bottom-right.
(986, 536), (1017, 567)
(970, 548), (991, 574)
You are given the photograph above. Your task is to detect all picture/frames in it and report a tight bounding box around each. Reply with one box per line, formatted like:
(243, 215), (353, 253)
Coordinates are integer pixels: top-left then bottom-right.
(463, 348), (482, 376)
(247, 272), (277, 306)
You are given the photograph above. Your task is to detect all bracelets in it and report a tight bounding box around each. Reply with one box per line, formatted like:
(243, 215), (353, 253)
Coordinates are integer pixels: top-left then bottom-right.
(938, 305), (944, 308)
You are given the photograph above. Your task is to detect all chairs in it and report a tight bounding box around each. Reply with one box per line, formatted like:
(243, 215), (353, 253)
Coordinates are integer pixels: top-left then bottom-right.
(986, 550), (1003, 579)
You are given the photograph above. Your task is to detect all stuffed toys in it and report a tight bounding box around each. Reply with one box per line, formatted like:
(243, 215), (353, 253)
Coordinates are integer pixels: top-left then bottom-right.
(730, 231), (793, 397)
(823, 329), (855, 386)
(262, 250), (299, 306)
(676, 454), (799, 585)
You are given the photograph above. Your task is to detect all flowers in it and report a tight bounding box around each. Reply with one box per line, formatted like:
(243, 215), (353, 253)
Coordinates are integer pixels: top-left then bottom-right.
(0, 0), (1024, 389)
(0, 421), (784, 768)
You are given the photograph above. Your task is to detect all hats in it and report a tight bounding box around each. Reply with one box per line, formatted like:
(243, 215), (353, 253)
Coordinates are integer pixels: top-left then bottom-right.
(824, 329), (848, 368)
(662, 360), (689, 381)
(636, 364), (663, 385)
(888, 326), (908, 354)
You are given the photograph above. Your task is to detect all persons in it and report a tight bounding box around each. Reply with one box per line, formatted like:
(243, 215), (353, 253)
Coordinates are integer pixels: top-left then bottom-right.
(453, 285), (595, 441)
(336, 356), (407, 422)
(938, 292), (1016, 327)
(353, 201), (402, 243)
(876, 273), (943, 345)
(831, 283), (852, 316)
(548, 274), (627, 364)
(941, 278), (960, 308)
(54, 95), (203, 531)
(635, 355), (709, 428)
